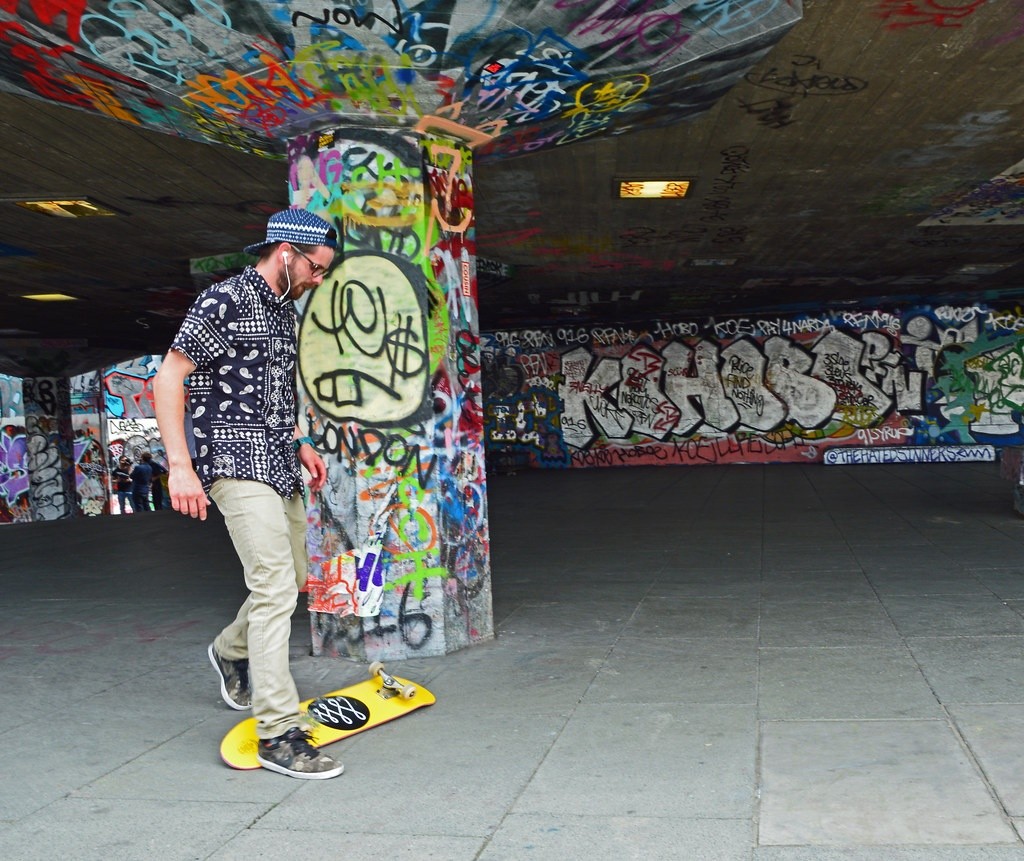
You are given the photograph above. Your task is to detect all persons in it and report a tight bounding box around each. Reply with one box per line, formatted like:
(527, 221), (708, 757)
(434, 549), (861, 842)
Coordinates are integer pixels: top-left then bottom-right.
(128, 453), (153, 513)
(149, 459), (169, 512)
(112, 456), (136, 514)
(151, 207), (344, 782)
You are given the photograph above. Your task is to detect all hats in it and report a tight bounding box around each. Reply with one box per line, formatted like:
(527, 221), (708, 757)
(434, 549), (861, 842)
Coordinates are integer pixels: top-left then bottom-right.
(243, 208), (337, 256)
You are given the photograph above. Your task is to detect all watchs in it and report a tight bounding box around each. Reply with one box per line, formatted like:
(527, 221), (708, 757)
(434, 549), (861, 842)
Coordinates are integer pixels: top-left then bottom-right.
(294, 436), (316, 450)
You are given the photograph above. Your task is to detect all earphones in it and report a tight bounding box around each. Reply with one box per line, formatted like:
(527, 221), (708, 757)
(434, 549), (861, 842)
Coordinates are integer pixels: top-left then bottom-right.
(282, 251), (288, 264)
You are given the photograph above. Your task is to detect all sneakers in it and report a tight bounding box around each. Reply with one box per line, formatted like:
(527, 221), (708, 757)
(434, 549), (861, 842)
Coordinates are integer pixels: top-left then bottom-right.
(208, 642), (253, 710)
(256, 729), (344, 779)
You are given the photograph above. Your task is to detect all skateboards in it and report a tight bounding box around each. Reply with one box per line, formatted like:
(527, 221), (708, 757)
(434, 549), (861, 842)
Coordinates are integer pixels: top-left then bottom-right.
(220, 658), (438, 771)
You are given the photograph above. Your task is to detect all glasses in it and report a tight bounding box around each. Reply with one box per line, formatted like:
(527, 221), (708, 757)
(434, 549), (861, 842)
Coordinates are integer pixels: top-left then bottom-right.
(291, 244), (329, 278)
